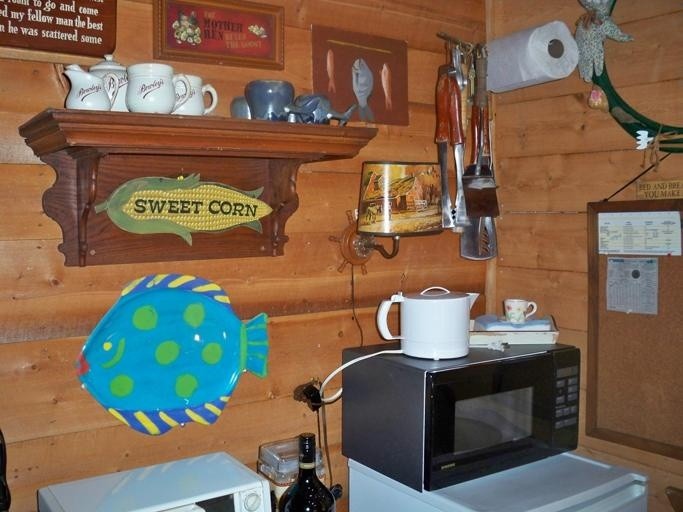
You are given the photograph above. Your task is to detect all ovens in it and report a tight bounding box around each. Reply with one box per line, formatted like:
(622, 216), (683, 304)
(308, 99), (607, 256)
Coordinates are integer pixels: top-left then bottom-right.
(341, 337), (582, 493)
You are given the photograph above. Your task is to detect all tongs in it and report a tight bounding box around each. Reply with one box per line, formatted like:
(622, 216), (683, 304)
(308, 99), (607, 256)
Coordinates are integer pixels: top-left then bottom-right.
(434, 63), (473, 229)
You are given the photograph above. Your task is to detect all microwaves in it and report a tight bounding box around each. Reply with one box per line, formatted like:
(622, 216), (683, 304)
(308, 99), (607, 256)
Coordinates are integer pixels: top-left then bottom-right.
(35, 451), (273, 512)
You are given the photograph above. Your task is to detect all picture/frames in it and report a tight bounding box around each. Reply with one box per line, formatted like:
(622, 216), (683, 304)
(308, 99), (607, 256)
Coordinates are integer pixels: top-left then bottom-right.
(151, 1), (285, 71)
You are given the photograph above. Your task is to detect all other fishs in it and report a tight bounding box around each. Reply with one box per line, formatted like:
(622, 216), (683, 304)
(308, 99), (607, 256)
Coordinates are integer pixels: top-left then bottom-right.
(351, 57), (375, 124)
(379, 61), (394, 110)
(325, 48), (337, 93)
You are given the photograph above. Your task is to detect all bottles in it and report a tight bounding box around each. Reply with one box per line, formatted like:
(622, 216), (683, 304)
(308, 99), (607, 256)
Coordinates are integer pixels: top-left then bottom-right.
(278, 433), (337, 512)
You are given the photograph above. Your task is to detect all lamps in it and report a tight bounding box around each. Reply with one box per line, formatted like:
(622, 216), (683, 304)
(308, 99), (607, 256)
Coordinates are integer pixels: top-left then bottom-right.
(329, 160), (445, 275)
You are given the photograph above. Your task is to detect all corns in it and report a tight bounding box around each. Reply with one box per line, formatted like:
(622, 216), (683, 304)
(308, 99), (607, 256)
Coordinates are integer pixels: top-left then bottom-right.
(93, 172), (275, 247)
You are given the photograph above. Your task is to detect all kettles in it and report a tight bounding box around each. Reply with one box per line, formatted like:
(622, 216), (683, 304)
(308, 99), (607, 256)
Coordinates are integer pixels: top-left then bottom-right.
(376, 285), (481, 361)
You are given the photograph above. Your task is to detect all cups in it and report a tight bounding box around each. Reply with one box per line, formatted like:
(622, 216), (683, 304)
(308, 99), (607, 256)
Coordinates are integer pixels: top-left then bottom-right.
(125, 63), (192, 116)
(170, 71), (219, 117)
(89, 54), (130, 113)
(504, 298), (537, 323)
(64, 64), (120, 113)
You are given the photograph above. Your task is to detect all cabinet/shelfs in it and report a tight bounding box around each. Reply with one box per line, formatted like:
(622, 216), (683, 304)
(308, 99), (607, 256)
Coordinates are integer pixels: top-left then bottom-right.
(19, 109), (378, 268)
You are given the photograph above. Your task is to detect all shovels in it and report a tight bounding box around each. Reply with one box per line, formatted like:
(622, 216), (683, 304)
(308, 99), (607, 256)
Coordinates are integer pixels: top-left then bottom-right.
(460, 41), (500, 261)
